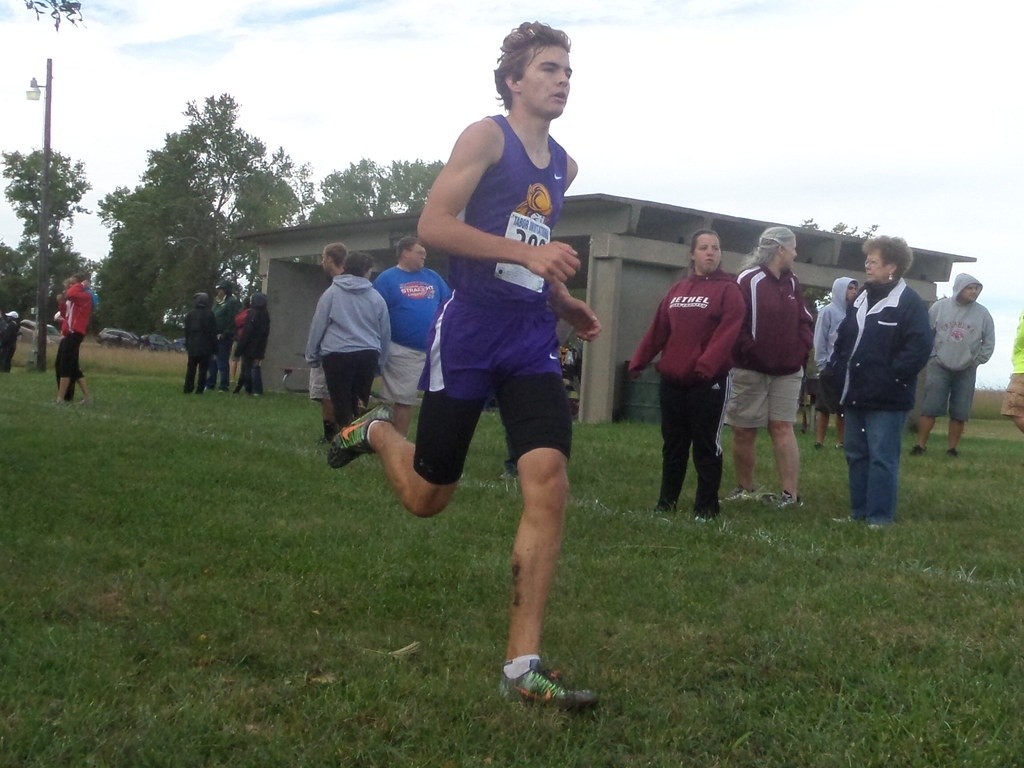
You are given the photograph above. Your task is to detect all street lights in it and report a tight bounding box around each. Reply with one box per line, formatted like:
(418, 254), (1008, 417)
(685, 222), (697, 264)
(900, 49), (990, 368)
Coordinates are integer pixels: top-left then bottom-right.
(26, 58), (52, 371)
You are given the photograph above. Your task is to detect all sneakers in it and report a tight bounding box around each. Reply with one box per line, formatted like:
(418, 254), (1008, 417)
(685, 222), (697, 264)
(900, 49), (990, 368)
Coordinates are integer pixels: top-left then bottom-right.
(327, 402), (395, 469)
(496, 663), (600, 711)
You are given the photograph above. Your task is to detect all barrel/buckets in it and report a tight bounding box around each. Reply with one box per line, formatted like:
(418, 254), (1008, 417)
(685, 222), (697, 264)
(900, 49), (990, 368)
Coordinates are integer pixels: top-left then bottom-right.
(622, 360), (663, 425)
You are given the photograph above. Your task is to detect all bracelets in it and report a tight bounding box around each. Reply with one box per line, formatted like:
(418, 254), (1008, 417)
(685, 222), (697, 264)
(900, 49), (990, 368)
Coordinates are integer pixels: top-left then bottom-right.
(545, 281), (570, 305)
(81, 282), (85, 289)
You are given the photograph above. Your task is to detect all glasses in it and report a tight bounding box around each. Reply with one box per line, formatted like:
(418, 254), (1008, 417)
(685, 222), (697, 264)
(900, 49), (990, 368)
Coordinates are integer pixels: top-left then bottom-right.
(864, 258), (879, 268)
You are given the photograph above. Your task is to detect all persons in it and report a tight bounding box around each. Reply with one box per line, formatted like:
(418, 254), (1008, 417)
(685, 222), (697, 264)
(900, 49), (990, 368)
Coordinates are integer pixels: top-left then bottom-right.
(306, 237), (453, 442)
(812, 272), (1024, 456)
(0, 272), (94, 403)
(627, 229), (748, 523)
(724, 226), (814, 507)
(328, 19), (600, 713)
(824, 236), (934, 527)
(183, 281), (269, 397)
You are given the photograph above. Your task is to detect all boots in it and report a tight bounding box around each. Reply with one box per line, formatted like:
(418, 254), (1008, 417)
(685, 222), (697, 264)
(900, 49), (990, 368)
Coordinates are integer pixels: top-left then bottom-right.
(318, 420), (337, 445)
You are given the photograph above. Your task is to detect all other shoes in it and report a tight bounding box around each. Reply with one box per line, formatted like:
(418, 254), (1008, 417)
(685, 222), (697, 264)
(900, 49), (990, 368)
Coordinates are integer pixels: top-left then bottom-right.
(774, 489), (804, 508)
(946, 449), (959, 458)
(716, 484), (756, 504)
(814, 441), (824, 449)
(909, 445), (927, 457)
(835, 441), (844, 449)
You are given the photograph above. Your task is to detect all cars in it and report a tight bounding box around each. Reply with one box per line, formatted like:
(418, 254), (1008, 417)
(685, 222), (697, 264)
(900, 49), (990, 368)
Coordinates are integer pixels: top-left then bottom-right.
(18, 317), (187, 352)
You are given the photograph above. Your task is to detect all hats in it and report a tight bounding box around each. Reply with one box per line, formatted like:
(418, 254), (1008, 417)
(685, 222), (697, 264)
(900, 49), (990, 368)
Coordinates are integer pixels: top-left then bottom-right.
(4, 311), (19, 319)
